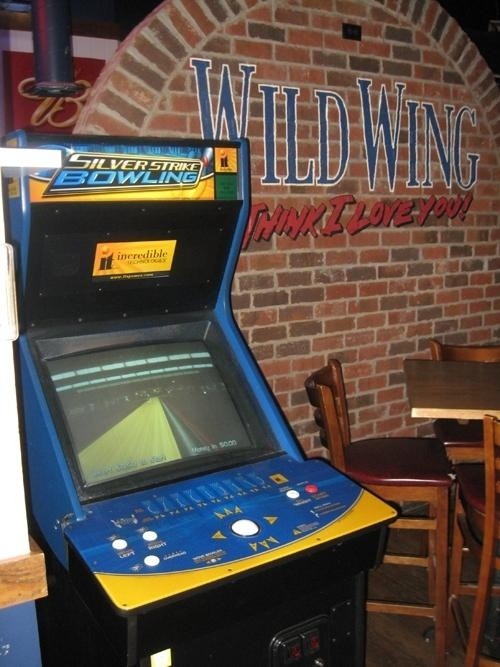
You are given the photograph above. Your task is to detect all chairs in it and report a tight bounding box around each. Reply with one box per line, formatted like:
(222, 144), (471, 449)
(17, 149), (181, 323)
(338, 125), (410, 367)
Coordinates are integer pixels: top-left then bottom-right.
(448, 414), (500, 665)
(304, 356), (455, 665)
(427, 338), (500, 468)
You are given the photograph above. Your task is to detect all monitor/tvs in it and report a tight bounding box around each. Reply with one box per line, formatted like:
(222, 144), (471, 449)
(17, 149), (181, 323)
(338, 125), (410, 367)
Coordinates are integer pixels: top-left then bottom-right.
(26, 309), (286, 503)
